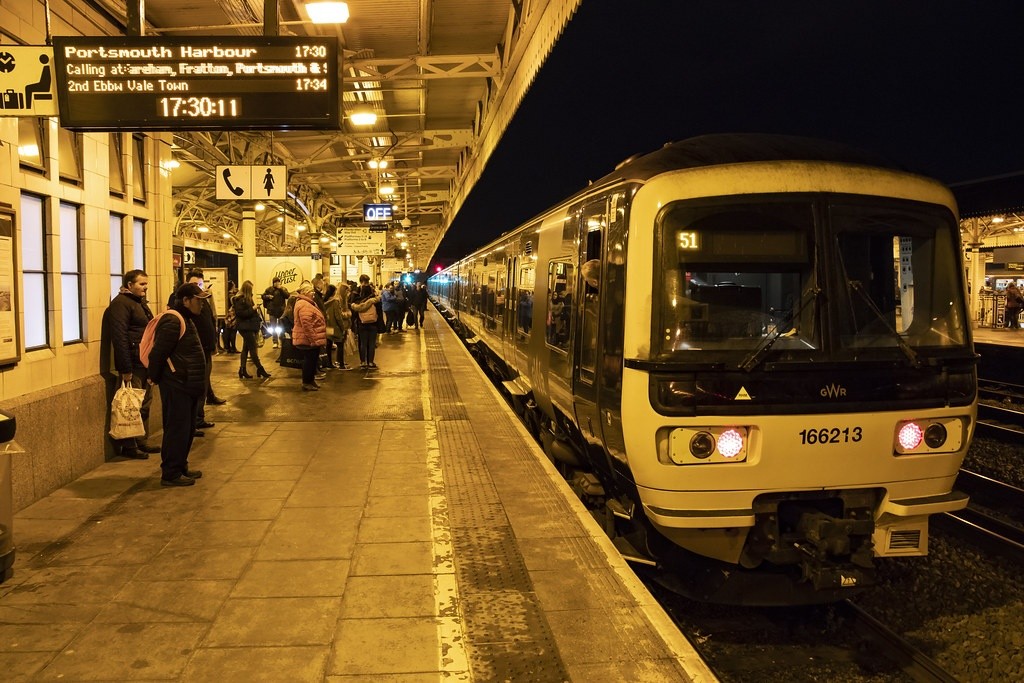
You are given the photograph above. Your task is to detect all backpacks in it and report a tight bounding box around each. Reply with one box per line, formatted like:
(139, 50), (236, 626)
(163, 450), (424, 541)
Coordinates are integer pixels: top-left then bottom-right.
(139, 309), (187, 371)
(225, 295), (237, 329)
(358, 304), (378, 323)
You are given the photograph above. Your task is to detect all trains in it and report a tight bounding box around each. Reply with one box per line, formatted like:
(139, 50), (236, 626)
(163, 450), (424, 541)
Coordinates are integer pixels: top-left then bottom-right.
(424, 130), (981, 605)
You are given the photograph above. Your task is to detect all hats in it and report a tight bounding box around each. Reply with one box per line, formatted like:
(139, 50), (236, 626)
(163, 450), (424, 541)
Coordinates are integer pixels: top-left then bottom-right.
(175, 283), (212, 300)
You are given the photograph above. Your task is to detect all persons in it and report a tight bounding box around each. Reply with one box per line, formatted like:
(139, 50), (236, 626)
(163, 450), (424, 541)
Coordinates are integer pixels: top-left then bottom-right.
(218, 281), (240, 353)
(280, 273), (430, 391)
(1002, 282), (1024, 327)
(232, 280), (272, 378)
(262, 277), (290, 348)
(108, 270), (162, 458)
(167, 281), (181, 310)
(472, 285), (572, 345)
(148, 283), (212, 485)
(185, 270), (226, 437)
(582, 259), (599, 289)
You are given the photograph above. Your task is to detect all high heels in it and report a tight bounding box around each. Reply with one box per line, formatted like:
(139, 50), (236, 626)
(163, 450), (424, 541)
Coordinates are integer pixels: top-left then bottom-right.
(256, 365), (271, 377)
(238, 367), (254, 379)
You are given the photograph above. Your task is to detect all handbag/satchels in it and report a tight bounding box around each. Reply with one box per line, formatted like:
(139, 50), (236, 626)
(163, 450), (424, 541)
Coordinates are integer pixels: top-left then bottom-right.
(109, 380), (147, 439)
(343, 329), (357, 356)
(255, 328), (264, 347)
(325, 326), (334, 335)
(280, 337), (306, 369)
(406, 309), (415, 326)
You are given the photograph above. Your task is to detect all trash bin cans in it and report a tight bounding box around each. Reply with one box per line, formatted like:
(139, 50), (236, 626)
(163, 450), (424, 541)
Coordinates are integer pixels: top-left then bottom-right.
(0, 409), (25, 574)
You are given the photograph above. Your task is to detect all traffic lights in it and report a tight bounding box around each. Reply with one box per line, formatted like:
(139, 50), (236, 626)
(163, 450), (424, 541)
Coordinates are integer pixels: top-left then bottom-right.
(436, 265), (441, 272)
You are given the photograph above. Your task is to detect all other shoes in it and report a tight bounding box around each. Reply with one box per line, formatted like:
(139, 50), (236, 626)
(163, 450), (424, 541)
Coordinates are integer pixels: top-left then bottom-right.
(121, 450), (149, 459)
(138, 445), (161, 453)
(273, 342), (278, 348)
(383, 320), (425, 334)
(211, 341), (240, 354)
(180, 465), (203, 479)
(194, 417), (215, 428)
(194, 428), (205, 437)
(304, 360), (378, 392)
(161, 472), (195, 486)
(206, 395), (226, 404)
